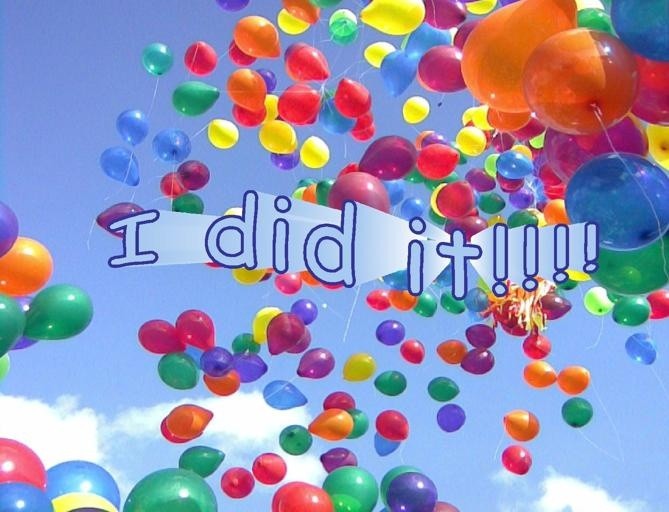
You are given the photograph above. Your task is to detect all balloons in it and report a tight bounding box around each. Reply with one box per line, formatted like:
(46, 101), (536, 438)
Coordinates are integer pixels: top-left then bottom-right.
(1, 2), (669, 512)
